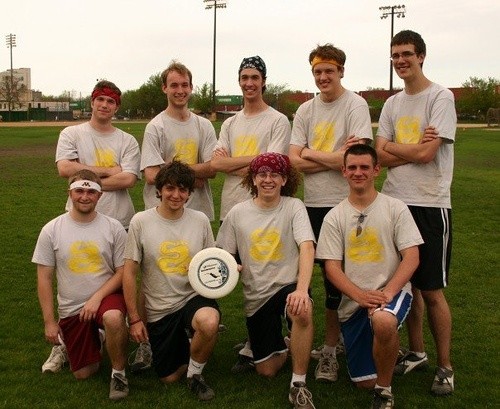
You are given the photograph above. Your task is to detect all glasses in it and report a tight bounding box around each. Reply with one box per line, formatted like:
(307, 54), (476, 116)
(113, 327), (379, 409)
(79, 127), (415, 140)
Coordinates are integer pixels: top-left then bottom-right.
(256, 171), (283, 177)
(390, 52), (416, 61)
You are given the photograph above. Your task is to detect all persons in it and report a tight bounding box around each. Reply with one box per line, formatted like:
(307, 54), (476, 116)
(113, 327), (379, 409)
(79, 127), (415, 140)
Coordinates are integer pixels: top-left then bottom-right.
(31, 169), (130, 400)
(139, 58), (227, 344)
(41, 79), (142, 375)
(210, 56), (291, 359)
(314, 143), (425, 409)
(122, 154), (243, 401)
(215, 152), (318, 409)
(375, 30), (457, 396)
(289, 42), (374, 384)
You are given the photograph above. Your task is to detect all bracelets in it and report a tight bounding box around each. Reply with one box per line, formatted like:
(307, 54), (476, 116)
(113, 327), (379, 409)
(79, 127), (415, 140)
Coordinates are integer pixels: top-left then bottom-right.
(129, 318), (141, 325)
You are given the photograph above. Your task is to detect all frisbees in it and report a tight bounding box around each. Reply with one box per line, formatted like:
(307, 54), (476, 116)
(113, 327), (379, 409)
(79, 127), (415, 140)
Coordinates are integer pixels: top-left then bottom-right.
(188, 247), (239, 299)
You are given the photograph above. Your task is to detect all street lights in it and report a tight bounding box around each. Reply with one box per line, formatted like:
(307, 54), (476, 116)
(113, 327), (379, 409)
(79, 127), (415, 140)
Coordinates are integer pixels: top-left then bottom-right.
(203, 0), (227, 122)
(379, 5), (406, 96)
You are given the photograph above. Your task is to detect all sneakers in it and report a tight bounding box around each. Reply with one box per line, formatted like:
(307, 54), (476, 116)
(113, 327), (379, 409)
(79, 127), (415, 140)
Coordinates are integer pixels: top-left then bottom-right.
(41, 345), (68, 374)
(185, 374), (215, 400)
(109, 373), (129, 400)
(394, 352), (429, 376)
(311, 338), (344, 358)
(314, 353), (339, 382)
(430, 366), (454, 395)
(289, 381), (316, 409)
(370, 388), (394, 408)
